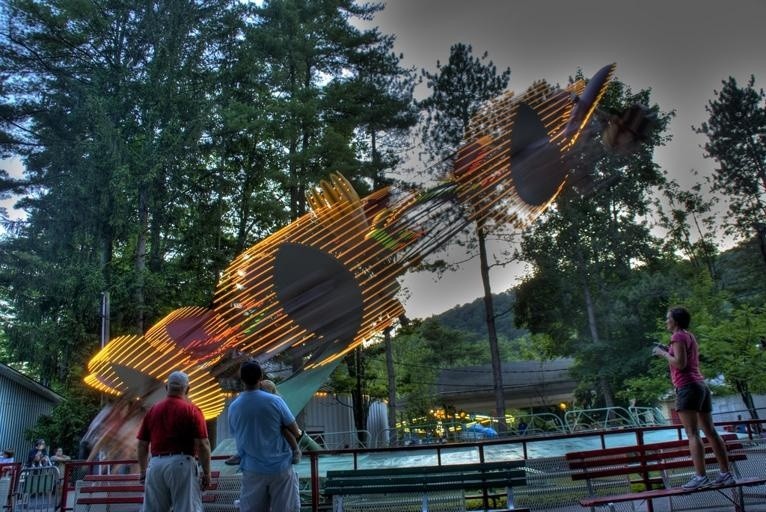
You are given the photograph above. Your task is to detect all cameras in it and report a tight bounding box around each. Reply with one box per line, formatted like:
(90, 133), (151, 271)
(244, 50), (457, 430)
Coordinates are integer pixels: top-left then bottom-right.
(652, 342), (668, 358)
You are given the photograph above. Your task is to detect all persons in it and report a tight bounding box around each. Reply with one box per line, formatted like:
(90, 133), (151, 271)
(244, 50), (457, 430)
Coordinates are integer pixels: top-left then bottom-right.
(135, 371), (212, 512)
(651, 307), (736, 491)
(0, 448), (16, 463)
(735, 415), (747, 432)
(27, 439), (71, 507)
(224, 360), (302, 512)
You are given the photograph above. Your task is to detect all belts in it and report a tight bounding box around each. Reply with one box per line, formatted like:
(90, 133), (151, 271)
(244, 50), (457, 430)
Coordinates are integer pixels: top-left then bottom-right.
(152, 452), (194, 457)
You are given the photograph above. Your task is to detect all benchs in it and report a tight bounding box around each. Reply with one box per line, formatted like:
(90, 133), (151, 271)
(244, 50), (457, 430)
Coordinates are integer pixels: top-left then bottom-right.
(325, 457), (531, 511)
(565, 435), (766, 512)
(76, 469), (220, 510)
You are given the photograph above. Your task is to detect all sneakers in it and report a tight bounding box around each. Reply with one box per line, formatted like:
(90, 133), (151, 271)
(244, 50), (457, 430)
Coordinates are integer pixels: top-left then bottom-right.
(224, 455), (241, 465)
(291, 448), (302, 464)
(681, 471), (736, 491)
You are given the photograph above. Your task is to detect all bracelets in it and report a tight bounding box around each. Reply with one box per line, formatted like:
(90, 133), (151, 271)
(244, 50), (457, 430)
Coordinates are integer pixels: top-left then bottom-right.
(664, 352), (667, 356)
